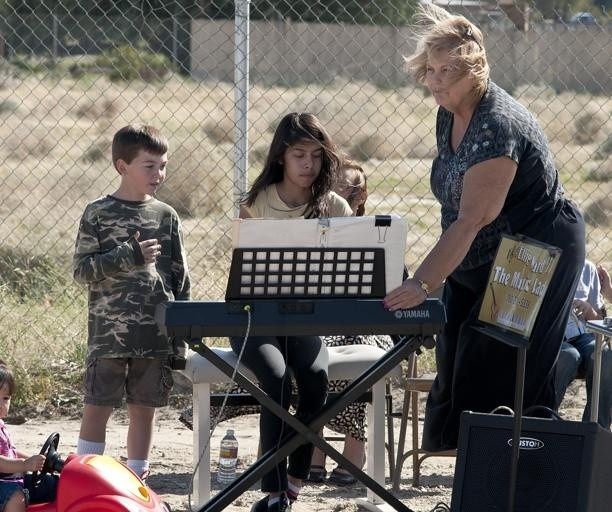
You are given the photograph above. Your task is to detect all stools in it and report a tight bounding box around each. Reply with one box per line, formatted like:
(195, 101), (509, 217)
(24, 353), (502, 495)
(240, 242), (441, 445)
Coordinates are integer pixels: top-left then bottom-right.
(184, 345), (403, 508)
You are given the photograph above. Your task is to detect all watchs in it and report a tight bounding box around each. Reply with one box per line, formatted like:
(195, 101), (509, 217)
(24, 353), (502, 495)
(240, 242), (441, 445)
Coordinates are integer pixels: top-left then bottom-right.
(413, 275), (429, 297)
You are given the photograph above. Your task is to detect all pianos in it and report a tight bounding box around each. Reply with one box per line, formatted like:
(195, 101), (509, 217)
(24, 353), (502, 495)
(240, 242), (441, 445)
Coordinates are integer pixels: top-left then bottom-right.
(154, 295), (450, 340)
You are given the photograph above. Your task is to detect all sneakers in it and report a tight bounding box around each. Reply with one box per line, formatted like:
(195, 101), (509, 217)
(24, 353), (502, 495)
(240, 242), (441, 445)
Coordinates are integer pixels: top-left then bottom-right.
(250, 491), (298, 512)
(268, 496), (290, 512)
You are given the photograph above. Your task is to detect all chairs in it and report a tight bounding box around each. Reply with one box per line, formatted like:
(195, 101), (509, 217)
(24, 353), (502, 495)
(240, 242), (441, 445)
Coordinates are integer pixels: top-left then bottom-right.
(390, 282), (459, 497)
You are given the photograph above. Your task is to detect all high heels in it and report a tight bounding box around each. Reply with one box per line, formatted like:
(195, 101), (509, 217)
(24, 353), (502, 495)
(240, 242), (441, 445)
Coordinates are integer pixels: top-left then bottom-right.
(308, 453), (327, 484)
(330, 455), (367, 485)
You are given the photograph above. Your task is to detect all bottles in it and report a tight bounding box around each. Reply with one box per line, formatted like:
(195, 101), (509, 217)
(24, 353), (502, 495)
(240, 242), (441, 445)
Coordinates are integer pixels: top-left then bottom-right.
(216, 430), (238, 484)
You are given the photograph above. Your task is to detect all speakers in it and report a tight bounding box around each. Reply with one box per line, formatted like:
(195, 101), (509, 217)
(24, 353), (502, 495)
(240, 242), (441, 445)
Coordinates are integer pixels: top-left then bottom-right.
(449, 410), (611, 512)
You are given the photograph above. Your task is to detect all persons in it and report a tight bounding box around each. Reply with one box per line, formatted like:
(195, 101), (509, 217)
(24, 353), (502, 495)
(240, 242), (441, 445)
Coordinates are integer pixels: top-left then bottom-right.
(382, 1), (587, 452)
(0, 358), (48, 511)
(226, 111), (362, 512)
(71, 122), (192, 484)
(598, 262), (612, 308)
(545, 257), (611, 430)
(306, 156), (409, 485)
